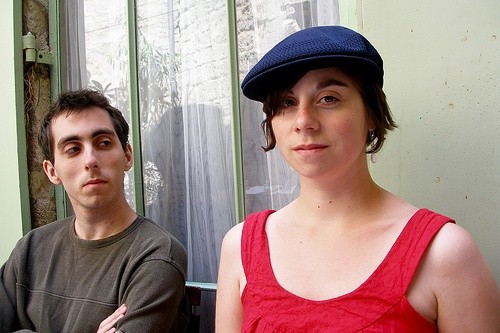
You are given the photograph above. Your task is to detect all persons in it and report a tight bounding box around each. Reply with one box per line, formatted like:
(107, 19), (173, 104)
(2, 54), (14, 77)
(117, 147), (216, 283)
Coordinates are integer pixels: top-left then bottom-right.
(0, 90), (188, 333)
(215, 26), (500, 333)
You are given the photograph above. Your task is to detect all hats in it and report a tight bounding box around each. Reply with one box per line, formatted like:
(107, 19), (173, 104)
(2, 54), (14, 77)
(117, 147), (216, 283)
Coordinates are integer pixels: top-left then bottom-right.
(241, 25), (384, 103)
